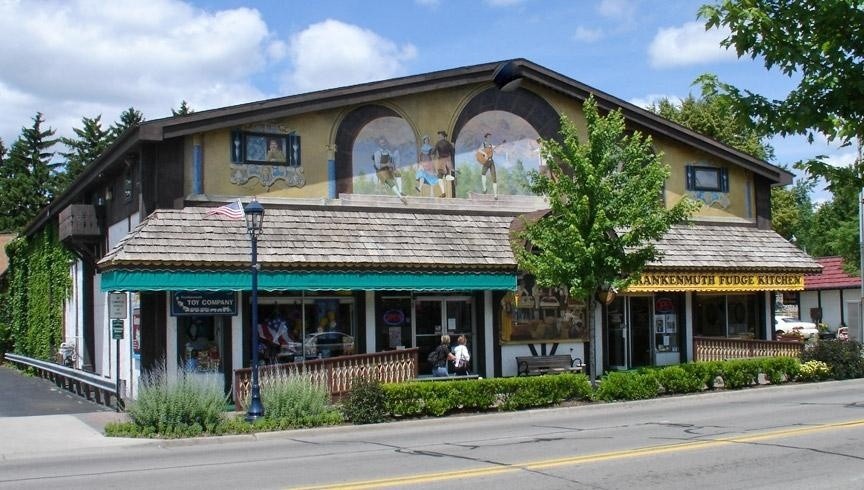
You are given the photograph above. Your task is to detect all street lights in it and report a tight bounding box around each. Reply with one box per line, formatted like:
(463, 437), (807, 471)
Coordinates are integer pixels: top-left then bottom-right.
(238, 195), (266, 422)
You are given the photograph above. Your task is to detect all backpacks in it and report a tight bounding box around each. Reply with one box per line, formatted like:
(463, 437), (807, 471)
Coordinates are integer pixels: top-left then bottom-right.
(427, 345), (446, 364)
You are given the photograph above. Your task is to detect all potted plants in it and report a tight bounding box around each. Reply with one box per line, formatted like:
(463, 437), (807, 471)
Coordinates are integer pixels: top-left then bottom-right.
(776, 330), (804, 343)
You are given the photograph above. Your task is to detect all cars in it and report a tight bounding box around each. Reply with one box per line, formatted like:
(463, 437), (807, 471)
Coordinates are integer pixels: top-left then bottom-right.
(280, 331), (355, 357)
(498, 317), (529, 339)
(774, 315), (818, 342)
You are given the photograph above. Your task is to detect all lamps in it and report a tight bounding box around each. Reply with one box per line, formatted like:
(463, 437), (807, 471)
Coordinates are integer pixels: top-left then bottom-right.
(105, 184), (113, 201)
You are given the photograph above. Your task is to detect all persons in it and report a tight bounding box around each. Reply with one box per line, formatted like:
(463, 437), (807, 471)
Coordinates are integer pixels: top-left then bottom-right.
(373, 135), (410, 205)
(478, 133), (497, 198)
(454, 334), (470, 375)
(267, 139), (284, 162)
(432, 130), (454, 196)
(415, 135), (438, 192)
(432, 334), (456, 377)
(184, 349), (200, 372)
(517, 280), (529, 296)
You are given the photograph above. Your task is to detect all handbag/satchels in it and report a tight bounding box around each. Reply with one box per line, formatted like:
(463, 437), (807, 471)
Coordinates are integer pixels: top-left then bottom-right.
(459, 359), (468, 371)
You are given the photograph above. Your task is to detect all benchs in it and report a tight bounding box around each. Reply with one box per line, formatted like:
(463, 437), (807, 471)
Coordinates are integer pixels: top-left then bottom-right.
(515, 355), (582, 377)
